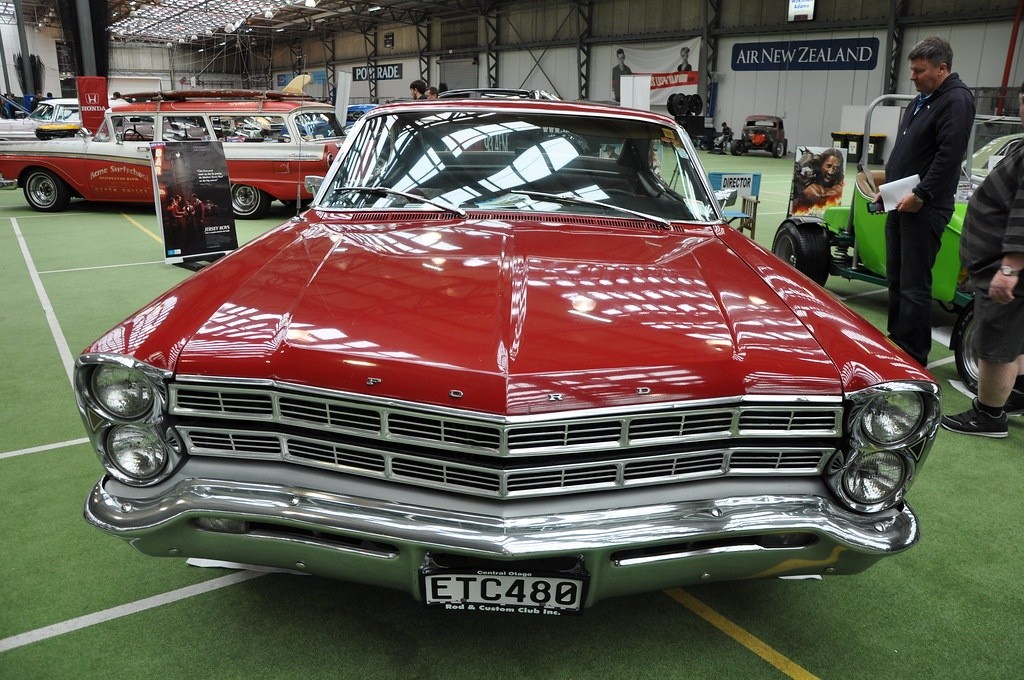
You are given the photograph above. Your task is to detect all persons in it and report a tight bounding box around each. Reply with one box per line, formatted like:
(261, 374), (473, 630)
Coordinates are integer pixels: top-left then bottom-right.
(940, 79), (1024, 438)
(166, 193), (205, 233)
(677, 46), (692, 73)
(602, 144), (620, 159)
(46, 93), (54, 100)
(0, 94), (15, 119)
(424, 86), (438, 99)
(108, 91), (130, 105)
(409, 80), (427, 99)
(872, 36), (977, 368)
(792, 148), (844, 215)
(721, 121), (733, 143)
(30, 90), (42, 112)
(611, 49), (631, 102)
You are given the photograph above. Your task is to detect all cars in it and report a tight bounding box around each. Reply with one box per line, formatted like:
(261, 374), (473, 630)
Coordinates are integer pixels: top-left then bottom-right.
(278, 104), (379, 142)
(730, 115), (788, 159)
(438, 86), (602, 156)
(35, 116), (205, 138)
(73, 97), (942, 618)
(0, 95), (140, 137)
(771, 94), (1024, 413)
(0, 102), (379, 221)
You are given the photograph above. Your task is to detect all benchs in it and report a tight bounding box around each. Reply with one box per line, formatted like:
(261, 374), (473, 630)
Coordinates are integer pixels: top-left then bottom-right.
(406, 165), (632, 198)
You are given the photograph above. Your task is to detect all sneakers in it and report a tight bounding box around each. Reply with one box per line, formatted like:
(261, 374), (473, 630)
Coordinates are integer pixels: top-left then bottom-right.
(1003, 389), (1024, 417)
(940, 398), (1008, 439)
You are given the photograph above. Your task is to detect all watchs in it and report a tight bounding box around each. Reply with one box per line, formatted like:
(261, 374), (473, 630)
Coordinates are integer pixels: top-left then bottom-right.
(912, 193), (925, 204)
(1000, 265), (1019, 277)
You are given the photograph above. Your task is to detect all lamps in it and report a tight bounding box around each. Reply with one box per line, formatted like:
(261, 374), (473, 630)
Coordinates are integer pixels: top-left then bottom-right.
(264, 8), (274, 19)
(166, 41), (174, 48)
(178, 38), (186, 44)
(223, 21), (236, 34)
(205, 27), (213, 37)
(305, 0), (317, 7)
(191, 34), (198, 39)
